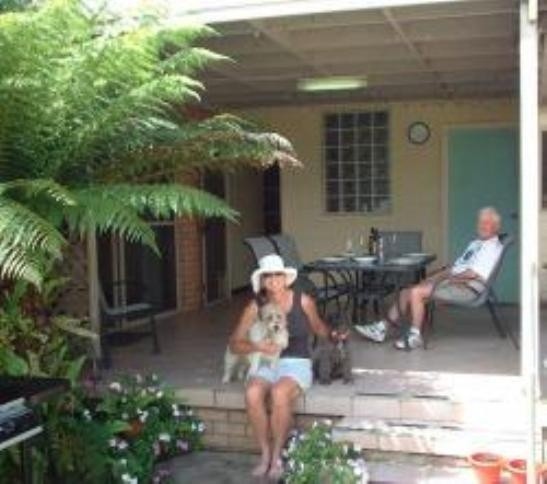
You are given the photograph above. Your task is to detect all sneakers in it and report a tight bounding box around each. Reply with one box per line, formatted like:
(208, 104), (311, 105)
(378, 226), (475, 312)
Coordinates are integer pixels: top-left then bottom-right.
(395, 331), (423, 349)
(354, 322), (387, 343)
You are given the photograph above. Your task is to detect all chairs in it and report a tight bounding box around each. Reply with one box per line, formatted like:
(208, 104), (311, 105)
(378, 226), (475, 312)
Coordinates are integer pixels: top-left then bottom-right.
(423, 231), (521, 352)
(359, 230), (429, 326)
(241, 235), (281, 268)
(97, 273), (159, 367)
(269, 233), (350, 320)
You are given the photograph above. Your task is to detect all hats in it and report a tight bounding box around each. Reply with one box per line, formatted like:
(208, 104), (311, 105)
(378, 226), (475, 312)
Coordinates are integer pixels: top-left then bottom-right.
(250, 254), (298, 293)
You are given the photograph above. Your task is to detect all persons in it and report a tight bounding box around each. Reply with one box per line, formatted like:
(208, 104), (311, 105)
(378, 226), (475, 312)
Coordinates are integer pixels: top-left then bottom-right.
(228, 253), (349, 482)
(354, 208), (506, 350)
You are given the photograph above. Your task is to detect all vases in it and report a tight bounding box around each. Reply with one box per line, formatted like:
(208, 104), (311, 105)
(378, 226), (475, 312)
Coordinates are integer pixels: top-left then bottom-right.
(467, 451), (506, 484)
(503, 459), (544, 484)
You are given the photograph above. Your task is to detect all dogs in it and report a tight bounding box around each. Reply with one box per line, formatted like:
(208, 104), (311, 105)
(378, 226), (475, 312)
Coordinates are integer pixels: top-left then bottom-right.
(221, 302), (289, 384)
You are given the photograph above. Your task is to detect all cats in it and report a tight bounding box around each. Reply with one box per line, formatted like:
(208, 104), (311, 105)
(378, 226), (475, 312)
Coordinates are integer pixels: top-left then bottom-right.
(307, 325), (351, 386)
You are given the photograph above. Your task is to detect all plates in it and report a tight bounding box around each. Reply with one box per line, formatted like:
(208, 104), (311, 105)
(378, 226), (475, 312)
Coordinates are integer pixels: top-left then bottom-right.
(320, 256), (345, 263)
(390, 257), (417, 266)
(354, 256), (376, 266)
(403, 254), (429, 256)
